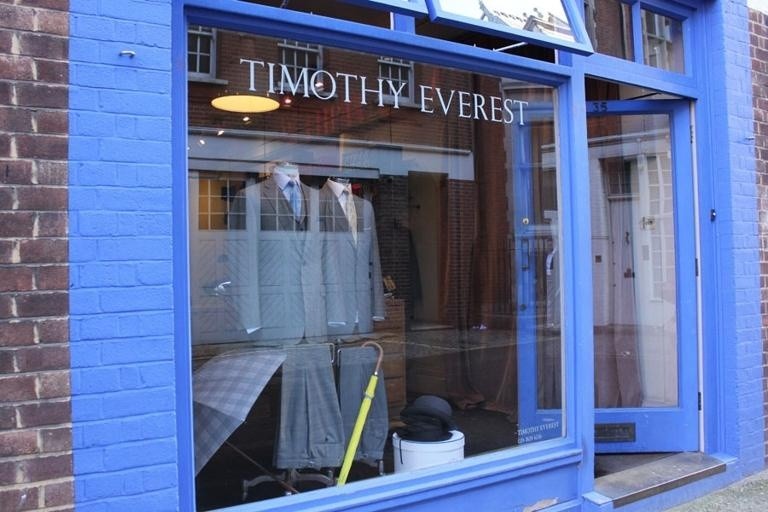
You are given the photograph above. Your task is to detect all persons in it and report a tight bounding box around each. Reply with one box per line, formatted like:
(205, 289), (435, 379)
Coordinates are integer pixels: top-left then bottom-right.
(226, 164), (385, 344)
